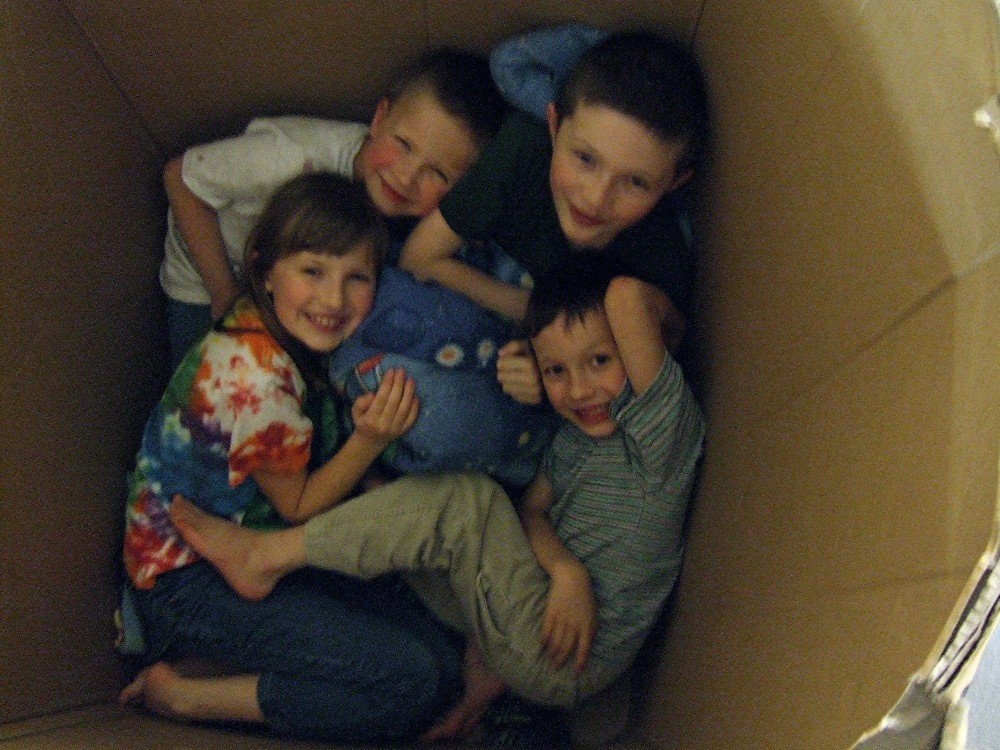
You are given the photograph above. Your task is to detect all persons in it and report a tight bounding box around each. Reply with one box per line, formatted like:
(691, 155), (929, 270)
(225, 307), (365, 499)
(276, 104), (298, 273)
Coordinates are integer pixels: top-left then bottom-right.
(166, 245), (704, 750)
(108, 26), (706, 750)
